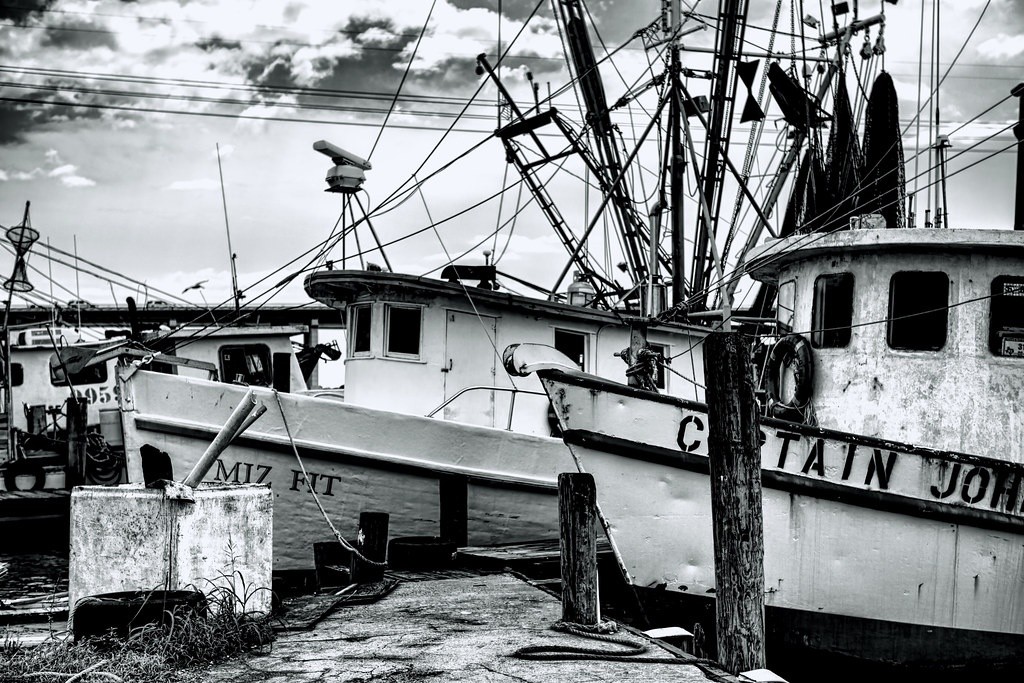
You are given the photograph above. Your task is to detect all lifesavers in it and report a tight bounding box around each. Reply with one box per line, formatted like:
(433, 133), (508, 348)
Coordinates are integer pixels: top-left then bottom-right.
(4, 458), (46, 491)
(764, 332), (815, 421)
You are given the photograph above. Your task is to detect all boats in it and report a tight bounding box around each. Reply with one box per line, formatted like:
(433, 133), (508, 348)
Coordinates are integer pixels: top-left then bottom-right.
(0, 0), (1024, 683)
